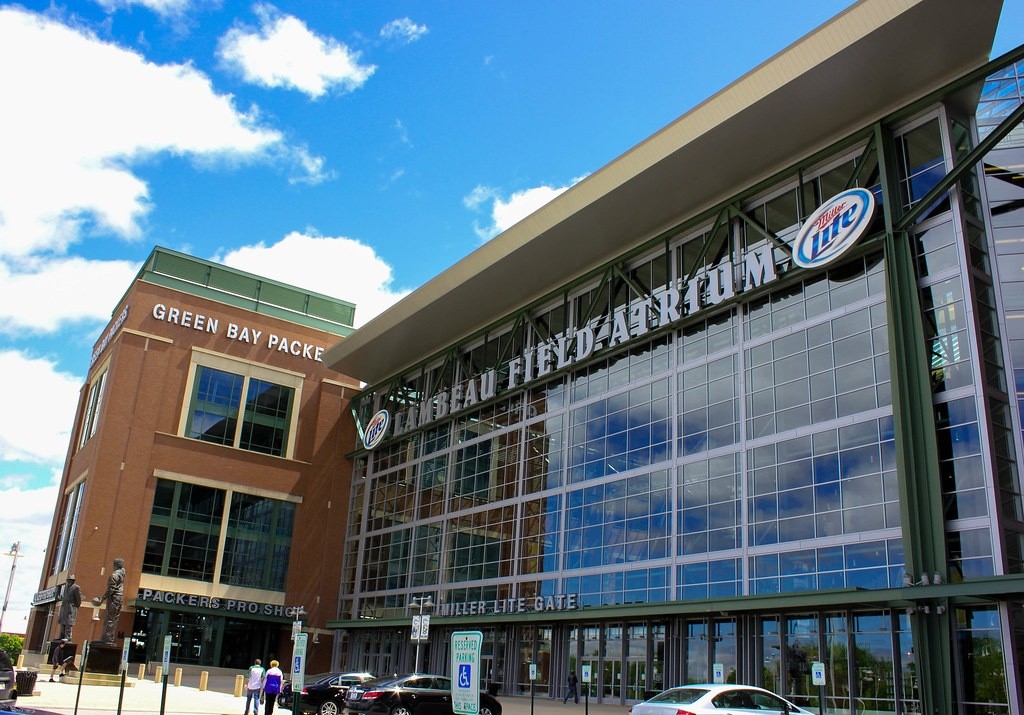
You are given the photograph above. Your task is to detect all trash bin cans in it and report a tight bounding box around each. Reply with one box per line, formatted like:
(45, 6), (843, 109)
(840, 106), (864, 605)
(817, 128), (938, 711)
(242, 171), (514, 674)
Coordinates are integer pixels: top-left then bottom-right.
(15, 670), (40, 696)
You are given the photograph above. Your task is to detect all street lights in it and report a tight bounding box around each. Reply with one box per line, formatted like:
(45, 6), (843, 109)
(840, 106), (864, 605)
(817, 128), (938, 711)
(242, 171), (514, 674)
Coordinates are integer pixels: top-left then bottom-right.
(290, 606), (307, 621)
(409, 595), (437, 674)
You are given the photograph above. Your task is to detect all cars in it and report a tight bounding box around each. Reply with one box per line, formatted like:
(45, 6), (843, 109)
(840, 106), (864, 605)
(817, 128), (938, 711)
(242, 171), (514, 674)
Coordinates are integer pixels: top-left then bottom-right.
(0, 649), (18, 710)
(340, 672), (503, 715)
(279, 672), (377, 715)
(627, 684), (816, 714)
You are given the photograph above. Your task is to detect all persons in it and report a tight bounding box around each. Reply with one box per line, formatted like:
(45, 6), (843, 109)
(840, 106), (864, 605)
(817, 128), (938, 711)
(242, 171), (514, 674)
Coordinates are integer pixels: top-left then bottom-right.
(563, 670), (579, 704)
(772, 643), (807, 694)
(243, 659), (266, 715)
(48, 641), (65, 682)
(262, 660), (283, 715)
(99, 558), (125, 643)
(58, 575), (82, 640)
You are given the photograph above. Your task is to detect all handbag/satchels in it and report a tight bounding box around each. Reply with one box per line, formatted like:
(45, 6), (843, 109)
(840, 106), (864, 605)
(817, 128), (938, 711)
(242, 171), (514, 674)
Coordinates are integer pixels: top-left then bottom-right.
(260, 690), (265, 704)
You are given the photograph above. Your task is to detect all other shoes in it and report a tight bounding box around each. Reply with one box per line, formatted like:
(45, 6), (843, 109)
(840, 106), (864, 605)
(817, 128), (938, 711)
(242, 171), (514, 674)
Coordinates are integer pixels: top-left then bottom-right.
(49, 679), (55, 682)
(59, 673), (65, 677)
